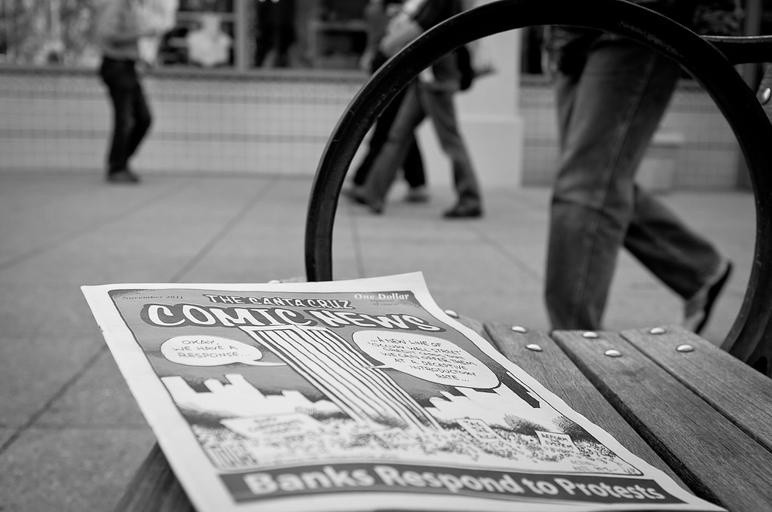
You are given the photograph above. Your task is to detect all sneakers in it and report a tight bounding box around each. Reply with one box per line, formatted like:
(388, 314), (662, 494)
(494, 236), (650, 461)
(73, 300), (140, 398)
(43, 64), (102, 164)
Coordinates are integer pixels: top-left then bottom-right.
(445, 197), (480, 216)
(683, 259), (732, 335)
(342, 184), (384, 212)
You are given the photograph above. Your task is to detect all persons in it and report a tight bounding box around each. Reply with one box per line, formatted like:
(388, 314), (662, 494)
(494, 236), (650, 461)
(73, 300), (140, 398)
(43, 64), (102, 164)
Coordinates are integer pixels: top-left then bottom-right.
(544, 6), (732, 335)
(338, 0), (429, 201)
(162, 0), (401, 71)
(341, 0), (483, 216)
(97, 1), (158, 181)
